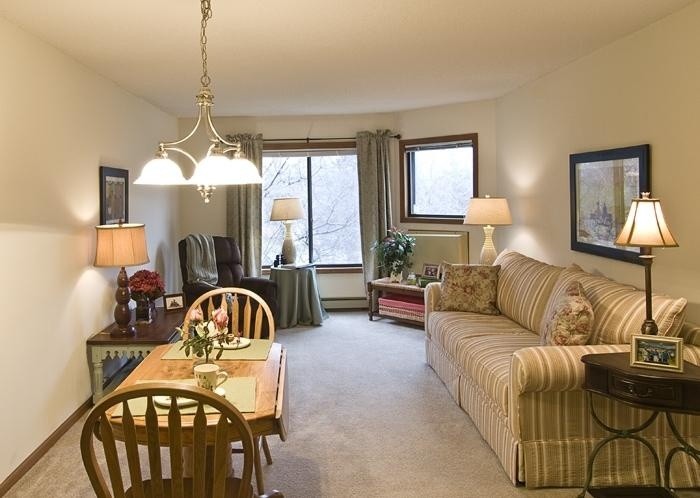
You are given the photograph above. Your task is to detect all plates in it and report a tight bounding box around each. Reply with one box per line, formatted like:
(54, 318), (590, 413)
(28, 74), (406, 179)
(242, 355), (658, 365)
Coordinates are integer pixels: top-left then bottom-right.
(153, 386), (226, 409)
(213, 336), (251, 349)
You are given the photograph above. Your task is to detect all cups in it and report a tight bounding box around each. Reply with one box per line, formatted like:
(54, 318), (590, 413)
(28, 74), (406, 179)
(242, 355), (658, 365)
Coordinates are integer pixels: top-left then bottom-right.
(193, 364), (228, 393)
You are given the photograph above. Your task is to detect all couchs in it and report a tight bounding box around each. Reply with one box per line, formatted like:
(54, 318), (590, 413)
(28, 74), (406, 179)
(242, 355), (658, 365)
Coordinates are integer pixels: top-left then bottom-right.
(424, 249), (699, 490)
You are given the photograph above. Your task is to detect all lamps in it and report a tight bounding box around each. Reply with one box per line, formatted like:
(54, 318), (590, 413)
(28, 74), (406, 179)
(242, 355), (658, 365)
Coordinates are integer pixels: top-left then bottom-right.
(462, 195), (512, 264)
(270, 197), (304, 264)
(131, 1), (263, 204)
(93, 223), (149, 338)
(615, 192), (678, 332)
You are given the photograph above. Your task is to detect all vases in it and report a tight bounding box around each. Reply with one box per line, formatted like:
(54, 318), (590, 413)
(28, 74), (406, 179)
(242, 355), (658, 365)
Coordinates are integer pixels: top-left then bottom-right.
(136, 300), (156, 324)
(390, 268), (402, 282)
(192, 359), (214, 373)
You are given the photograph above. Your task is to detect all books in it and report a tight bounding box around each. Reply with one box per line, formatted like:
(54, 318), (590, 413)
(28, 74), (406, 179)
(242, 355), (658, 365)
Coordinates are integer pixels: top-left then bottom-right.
(281, 263), (296, 268)
(418, 277), (435, 287)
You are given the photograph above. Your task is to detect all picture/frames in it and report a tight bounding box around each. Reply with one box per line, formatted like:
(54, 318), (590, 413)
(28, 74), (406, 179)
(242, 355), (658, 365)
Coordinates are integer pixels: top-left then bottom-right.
(630, 334), (684, 374)
(100, 166), (129, 225)
(422, 263), (441, 280)
(570, 145), (650, 265)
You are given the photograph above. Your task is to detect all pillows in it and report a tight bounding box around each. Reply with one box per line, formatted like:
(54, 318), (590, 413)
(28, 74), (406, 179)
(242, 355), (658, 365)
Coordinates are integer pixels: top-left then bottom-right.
(435, 261), (501, 316)
(540, 279), (599, 348)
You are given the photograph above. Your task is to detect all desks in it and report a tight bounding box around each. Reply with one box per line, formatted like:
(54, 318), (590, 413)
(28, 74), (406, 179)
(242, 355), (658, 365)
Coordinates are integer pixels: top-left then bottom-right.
(96, 342), (289, 477)
(87, 307), (188, 407)
(367, 277), (424, 329)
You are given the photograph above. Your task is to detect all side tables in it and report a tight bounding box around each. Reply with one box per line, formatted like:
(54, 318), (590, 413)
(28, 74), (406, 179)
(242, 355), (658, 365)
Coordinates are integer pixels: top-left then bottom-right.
(579, 354), (699, 498)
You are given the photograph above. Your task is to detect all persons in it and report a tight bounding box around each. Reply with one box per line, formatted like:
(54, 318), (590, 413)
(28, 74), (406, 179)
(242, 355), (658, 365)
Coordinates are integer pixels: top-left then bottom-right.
(642, 347), (671, 364)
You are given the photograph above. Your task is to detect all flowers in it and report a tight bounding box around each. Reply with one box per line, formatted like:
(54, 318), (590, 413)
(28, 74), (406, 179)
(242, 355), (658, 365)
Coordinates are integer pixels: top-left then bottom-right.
(174, 305), (243, 365)
(371, 227), (415, 271)
(128, 270), (165, 302)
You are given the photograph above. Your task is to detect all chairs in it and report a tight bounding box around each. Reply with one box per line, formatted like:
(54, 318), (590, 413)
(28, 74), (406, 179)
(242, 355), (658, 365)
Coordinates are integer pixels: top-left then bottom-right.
(178, 235), (279, 337)
(80, 382), (282, 498)
(182, 288), (272, 493)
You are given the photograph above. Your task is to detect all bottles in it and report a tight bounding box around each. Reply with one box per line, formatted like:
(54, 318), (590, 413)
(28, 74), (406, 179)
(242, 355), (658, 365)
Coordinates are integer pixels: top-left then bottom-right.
(406, 271), (417, 285)
(273, 254), (287, 268)
